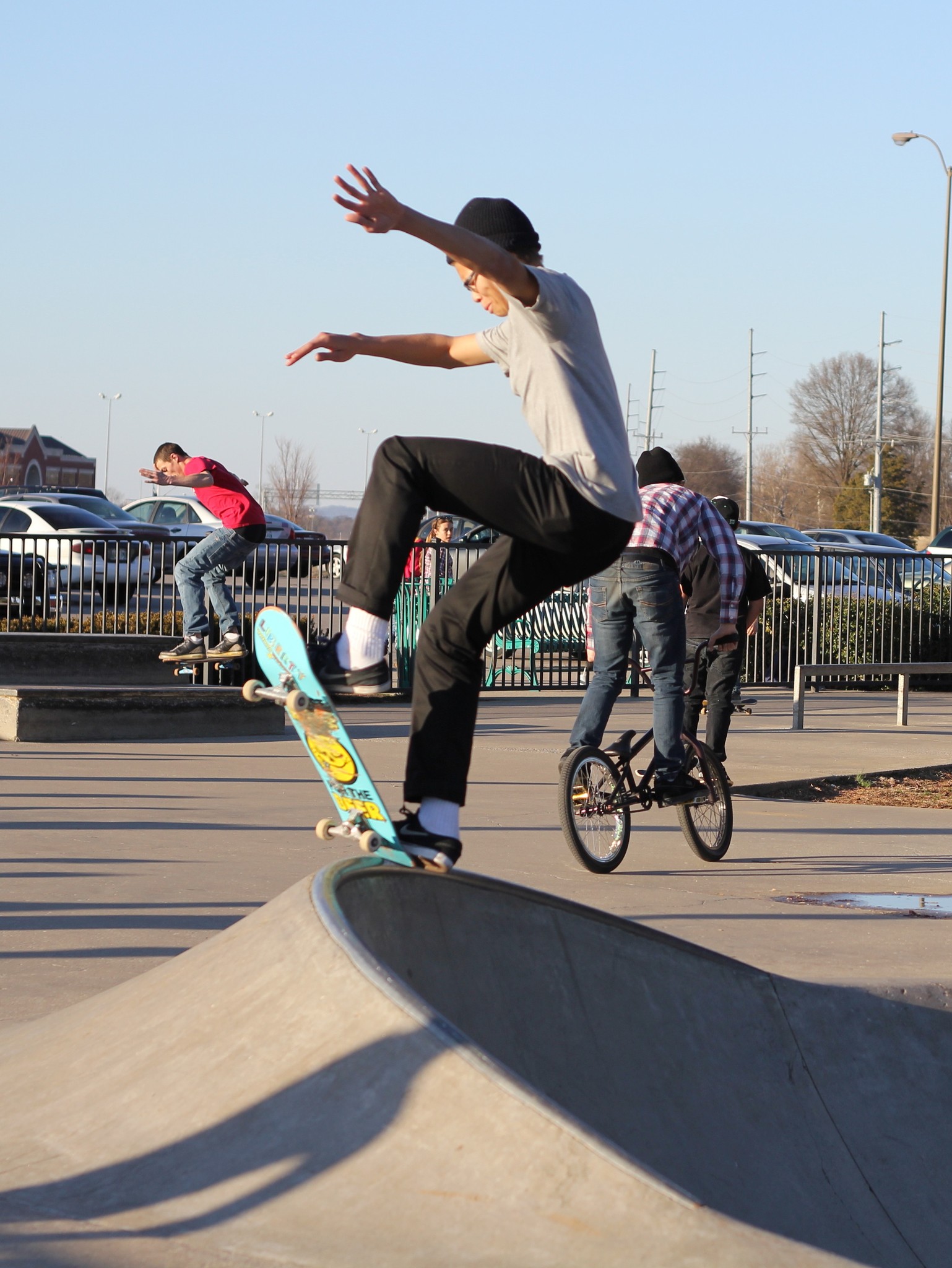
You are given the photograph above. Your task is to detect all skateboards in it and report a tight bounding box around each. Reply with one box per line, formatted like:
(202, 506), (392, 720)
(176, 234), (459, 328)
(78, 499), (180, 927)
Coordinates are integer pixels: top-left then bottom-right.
(162, 650), (249, 676)
(242, 605), (417, 871)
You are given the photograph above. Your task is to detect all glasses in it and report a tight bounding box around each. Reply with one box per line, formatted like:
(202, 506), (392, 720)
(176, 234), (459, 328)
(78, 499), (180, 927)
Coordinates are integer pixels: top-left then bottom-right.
(463, 271), (475, 291)
(435, 515), (453, 529)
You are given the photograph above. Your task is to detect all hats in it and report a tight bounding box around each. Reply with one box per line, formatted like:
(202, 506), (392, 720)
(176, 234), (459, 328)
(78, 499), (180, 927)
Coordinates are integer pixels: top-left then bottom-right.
(709, 495), (739, 532)
(636, 447), (685, 489)
(445, 197), (541, 266)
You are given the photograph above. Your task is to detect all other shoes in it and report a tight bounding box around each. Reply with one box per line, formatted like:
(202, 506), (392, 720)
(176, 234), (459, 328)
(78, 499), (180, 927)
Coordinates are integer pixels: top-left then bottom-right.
(558, 747), (594, 804)
(699, 763), (733, 789)
(654, 770), (710, 808)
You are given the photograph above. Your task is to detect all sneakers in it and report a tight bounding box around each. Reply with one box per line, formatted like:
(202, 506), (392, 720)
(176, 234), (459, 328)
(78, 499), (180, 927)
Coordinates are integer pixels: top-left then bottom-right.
(391, 806), (462, 874)
(158, 636), (207, 659)
(206, 635), (248, 658)
(310, 631), (391, 695)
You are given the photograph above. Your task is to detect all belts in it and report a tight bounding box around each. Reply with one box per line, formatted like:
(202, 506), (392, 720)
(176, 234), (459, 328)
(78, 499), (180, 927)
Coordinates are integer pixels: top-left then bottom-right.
(616, 554), (660, 564)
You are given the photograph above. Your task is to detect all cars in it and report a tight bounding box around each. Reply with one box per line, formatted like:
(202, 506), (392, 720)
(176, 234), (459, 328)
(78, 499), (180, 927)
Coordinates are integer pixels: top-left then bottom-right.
(0, 492), (171, 584)
(488, 520), (952, 657)
(1, 499), (155, 606)
(109, 494), (330, 590)
(1, 549), (68, 619)
(415, 514), (502, 583)
(316, 543), (349, 579)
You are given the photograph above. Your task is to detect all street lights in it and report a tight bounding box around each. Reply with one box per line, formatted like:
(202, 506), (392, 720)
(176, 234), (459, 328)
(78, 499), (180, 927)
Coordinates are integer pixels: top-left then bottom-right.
(251, 410), (274, 508)
(890, 129), (952, 545)
(357, 427), (378, 496)
(97, 392), (122, 498)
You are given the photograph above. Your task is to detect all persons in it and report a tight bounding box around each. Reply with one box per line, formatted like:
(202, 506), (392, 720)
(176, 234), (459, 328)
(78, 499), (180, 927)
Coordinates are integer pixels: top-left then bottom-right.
(285, 164), (644, 872)
(138, 443), (267, 659)
(680, 494), (773, 785)
(404, 516), (453, 595)
(559, 447), (747, 804)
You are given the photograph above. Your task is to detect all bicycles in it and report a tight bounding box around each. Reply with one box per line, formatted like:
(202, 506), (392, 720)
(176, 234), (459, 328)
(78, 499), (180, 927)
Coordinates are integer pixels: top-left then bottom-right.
(555, 630), (744, 874)
